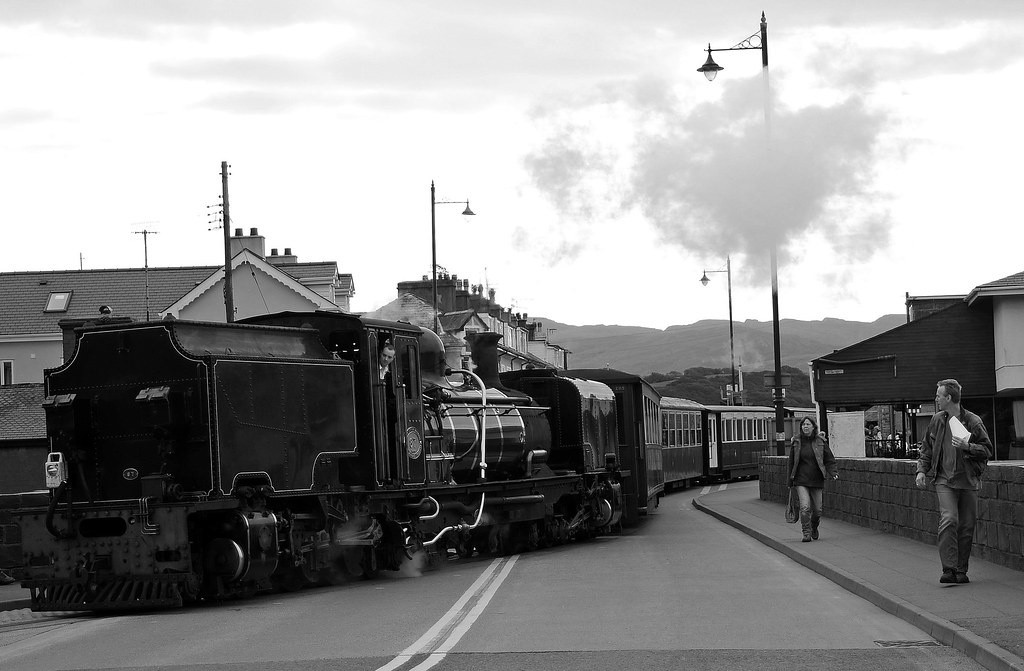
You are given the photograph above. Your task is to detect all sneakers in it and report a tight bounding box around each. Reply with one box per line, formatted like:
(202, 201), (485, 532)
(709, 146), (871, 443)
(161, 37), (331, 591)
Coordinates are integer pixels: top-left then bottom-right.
(956, 573), (969, 586)
(939, 568), (957, 586)
(811, 528), (819, 540)
(802, 533), (812, 542)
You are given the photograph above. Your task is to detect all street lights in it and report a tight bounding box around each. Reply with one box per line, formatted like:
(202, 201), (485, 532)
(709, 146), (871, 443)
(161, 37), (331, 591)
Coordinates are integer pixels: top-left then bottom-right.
(428, 181), (476, 334)
(701, 13), (787, 457)
(701, 257), (736, 408)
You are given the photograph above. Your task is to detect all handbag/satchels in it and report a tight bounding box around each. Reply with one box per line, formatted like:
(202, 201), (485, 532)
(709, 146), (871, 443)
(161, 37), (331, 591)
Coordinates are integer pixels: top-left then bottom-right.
(785, 488), (799, 524)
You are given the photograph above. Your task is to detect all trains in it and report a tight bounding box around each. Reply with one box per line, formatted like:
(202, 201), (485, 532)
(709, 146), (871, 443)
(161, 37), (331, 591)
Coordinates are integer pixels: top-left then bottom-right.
(20, 308), (830, 613)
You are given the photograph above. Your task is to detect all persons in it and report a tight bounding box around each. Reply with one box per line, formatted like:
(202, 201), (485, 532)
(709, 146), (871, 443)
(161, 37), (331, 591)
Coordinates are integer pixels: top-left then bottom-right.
(786, 416), (839, 542)
(916, 484), (919, 486)
(915, 380), (992, 586)
(859, 425), (922, 459)
